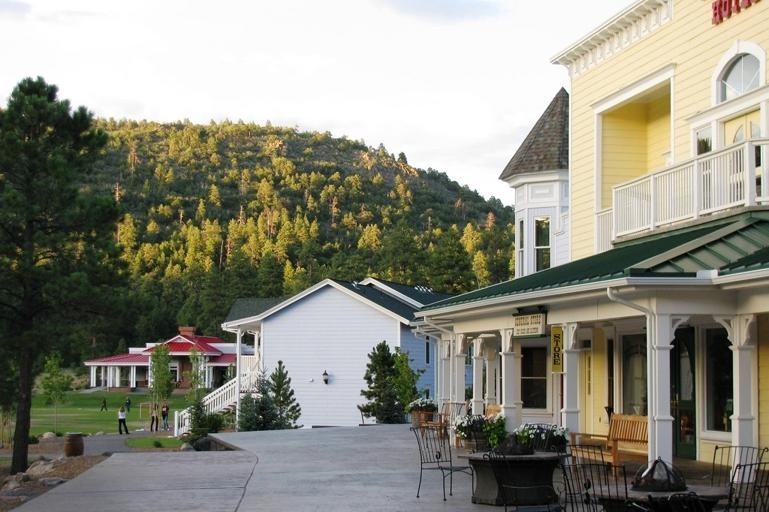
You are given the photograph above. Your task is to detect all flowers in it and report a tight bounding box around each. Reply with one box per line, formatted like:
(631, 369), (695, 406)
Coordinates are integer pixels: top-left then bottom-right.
(405, 398), (437, 412)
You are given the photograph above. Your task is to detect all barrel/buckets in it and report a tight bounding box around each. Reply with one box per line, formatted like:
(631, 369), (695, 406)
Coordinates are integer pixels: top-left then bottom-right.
(63, 434), (84, 457)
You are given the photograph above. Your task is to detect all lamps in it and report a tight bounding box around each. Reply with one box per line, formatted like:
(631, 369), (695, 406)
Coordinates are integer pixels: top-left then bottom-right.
(323, 371), (329, 384)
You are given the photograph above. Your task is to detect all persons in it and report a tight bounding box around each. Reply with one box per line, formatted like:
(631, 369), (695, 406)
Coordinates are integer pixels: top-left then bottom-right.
(100, 398), (109, 411)
(149, 402), (160, 432)
(118, 406), (131, 434)
(161, 399), (170, 431)
(125, 396), (131, 413)
